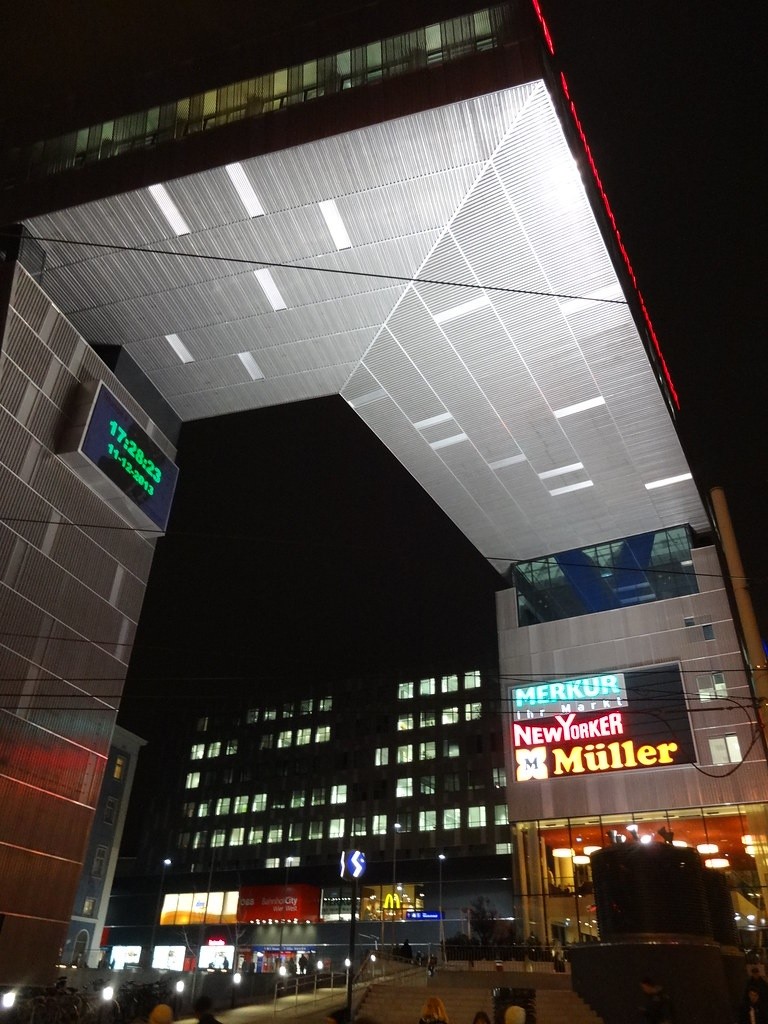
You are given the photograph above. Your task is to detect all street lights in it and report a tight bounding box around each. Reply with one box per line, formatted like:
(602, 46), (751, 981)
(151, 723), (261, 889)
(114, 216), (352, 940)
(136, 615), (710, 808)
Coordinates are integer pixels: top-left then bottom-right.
(437, 854), (446, 952)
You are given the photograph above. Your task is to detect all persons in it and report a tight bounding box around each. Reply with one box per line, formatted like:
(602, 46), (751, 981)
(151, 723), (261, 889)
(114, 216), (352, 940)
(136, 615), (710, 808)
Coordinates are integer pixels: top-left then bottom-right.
(505, 1006), (526, 1024)
(385, 940), (438, 978)
(744, 967), (768, 1024)
(249, 958), (255, 973)
(744, 988), (766, 1024)
(148, 1004), (174, 1024)
(222, 957), (229, 969)
(55, 949), (117, 969)
(274, 953), (320, 975)
(634, 975), (671, 1024)
(418, 995), (450, 1024)
(192, 995), (223, 1024)
(551, 952), (567, 974)
(473, 1011), (491, 1024)
(242, 959), (248, 972)
(208, 962), (215, 969)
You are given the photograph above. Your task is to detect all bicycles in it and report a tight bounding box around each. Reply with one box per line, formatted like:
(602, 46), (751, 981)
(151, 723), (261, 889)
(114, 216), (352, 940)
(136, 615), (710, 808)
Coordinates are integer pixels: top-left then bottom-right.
(0, 960), (179, 1024)
